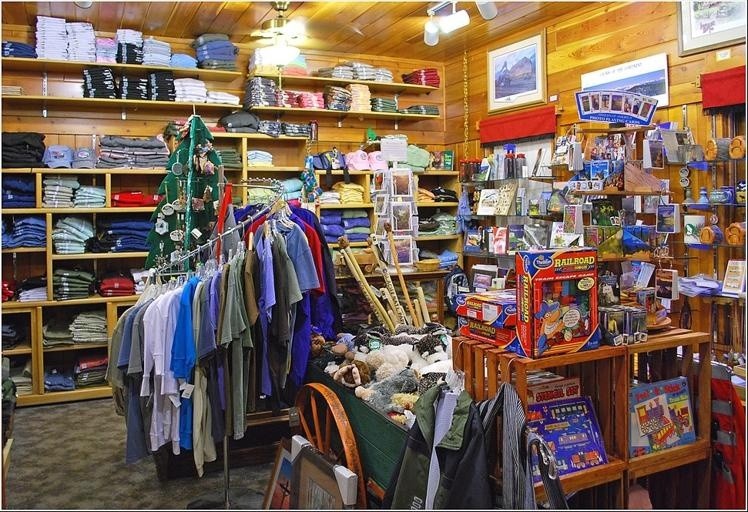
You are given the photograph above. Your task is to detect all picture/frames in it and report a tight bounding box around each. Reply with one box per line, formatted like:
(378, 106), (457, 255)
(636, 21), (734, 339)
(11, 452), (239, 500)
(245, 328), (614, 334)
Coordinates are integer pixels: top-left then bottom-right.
(486, 24), (549, 116)
(261, 436), (292, 510)
(673, 2), (747, 58)
(288, 435), (359, 510)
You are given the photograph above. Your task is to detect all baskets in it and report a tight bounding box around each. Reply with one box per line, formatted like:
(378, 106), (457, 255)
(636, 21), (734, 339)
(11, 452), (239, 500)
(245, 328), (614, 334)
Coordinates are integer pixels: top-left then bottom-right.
(414, 258), (440, 271)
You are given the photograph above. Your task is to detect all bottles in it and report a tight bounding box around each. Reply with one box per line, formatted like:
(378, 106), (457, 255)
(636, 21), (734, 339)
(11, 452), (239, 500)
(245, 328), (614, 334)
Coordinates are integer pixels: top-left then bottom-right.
(459, 159), (481, 182)
(504, 154), (516, 178)
(516, 154), (526, 177)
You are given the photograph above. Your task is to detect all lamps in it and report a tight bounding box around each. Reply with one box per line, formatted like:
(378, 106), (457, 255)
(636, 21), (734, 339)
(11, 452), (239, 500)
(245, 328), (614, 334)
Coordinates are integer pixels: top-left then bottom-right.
(261, 2), (291, 39)
(422, 2), (499, 48)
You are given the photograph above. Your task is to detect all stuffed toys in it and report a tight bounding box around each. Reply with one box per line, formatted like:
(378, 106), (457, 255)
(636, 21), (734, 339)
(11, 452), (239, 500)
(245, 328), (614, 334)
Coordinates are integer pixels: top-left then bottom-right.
(318, 318), (452, 431)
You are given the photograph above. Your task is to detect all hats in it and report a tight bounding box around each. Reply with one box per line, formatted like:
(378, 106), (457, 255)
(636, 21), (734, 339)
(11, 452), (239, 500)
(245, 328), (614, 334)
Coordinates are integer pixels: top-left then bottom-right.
(72, 147), (97, 169)
(368, 151), (388, 171)
(343, 150), (371, 171)
(42, 144), (73, 169)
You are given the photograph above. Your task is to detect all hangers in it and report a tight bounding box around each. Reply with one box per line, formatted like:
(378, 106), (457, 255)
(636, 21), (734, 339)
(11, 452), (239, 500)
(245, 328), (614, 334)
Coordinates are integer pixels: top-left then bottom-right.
(133, 184), (294, 306)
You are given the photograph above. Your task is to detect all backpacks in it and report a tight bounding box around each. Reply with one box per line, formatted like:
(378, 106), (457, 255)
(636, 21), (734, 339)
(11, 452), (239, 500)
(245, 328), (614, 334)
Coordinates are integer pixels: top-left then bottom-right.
(444, 264), (471, 317)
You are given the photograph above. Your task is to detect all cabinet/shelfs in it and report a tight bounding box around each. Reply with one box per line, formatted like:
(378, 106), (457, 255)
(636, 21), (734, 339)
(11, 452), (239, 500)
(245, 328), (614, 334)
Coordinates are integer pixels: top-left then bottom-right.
(2, 53), (463, 415)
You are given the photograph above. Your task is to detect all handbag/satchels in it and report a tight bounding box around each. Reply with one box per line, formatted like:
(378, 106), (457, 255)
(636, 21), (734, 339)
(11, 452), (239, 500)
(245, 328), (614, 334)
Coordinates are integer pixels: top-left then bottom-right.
(312, 146), (351, 188)
